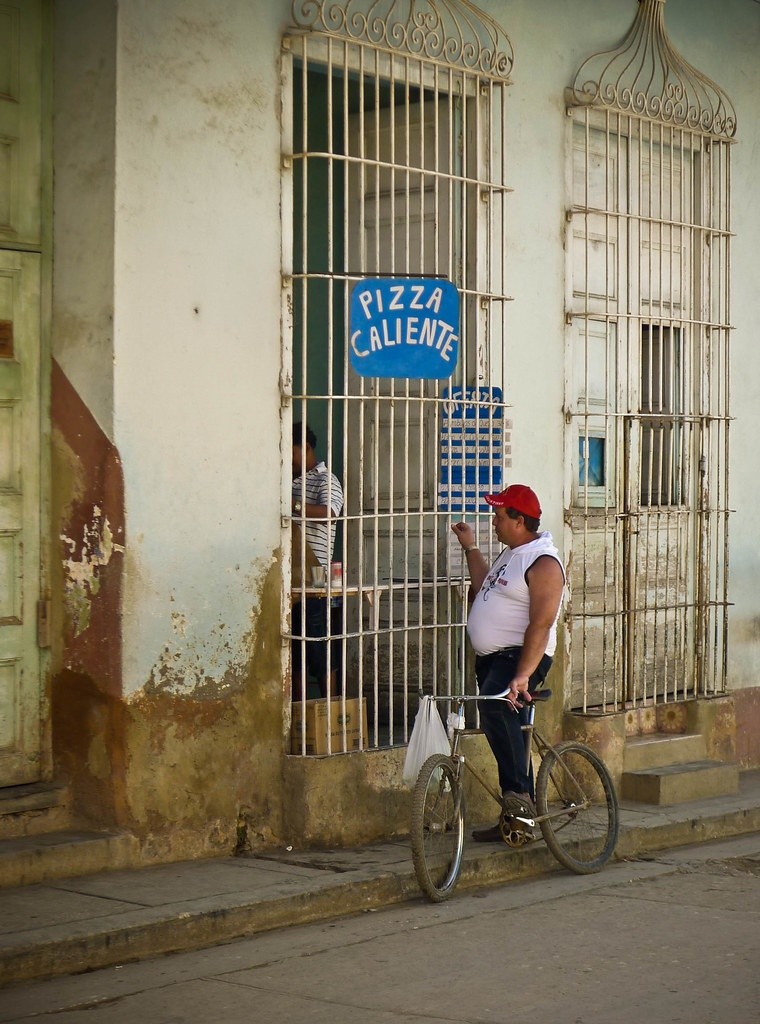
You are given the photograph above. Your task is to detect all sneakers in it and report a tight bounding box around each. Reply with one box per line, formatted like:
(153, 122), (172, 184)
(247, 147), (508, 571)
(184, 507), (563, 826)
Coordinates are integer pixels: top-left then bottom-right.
(471, 824), (533, 842)
(504, 790), (537, 819)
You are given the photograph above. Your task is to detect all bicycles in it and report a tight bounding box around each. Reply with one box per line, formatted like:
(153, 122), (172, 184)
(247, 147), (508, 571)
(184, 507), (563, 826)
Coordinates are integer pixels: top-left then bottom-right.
(412, 687), (620, 905)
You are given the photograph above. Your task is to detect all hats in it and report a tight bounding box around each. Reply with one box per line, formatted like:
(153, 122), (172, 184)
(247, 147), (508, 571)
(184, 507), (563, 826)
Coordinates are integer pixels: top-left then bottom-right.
(484, 483), (542, 519)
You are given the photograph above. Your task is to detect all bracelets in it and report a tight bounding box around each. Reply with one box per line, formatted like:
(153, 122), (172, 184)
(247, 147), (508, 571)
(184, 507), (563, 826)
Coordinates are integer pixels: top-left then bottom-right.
(463, 542), (481, 555)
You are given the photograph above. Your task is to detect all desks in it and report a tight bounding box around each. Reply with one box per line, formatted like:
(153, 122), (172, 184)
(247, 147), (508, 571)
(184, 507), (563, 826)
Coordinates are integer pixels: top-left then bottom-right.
(291, 578), (470, 633)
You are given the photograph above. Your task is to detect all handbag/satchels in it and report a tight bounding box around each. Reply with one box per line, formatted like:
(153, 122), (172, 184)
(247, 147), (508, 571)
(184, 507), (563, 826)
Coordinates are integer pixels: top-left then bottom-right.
(402, 695), (453, 792)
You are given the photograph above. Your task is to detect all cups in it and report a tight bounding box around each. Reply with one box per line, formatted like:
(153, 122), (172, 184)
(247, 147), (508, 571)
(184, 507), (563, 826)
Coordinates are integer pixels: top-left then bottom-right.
(329, 561), (342, 589)
(310, 566), (326, 588)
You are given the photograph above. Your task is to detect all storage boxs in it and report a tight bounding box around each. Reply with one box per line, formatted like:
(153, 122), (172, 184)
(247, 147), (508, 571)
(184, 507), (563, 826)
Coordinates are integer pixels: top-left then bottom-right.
(292, 694), (370, 755)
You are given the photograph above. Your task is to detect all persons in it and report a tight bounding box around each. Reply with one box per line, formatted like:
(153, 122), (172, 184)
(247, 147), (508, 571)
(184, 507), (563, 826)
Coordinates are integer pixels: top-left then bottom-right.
(289, 420), (345, 702)
(450, 483), (567, 844)
